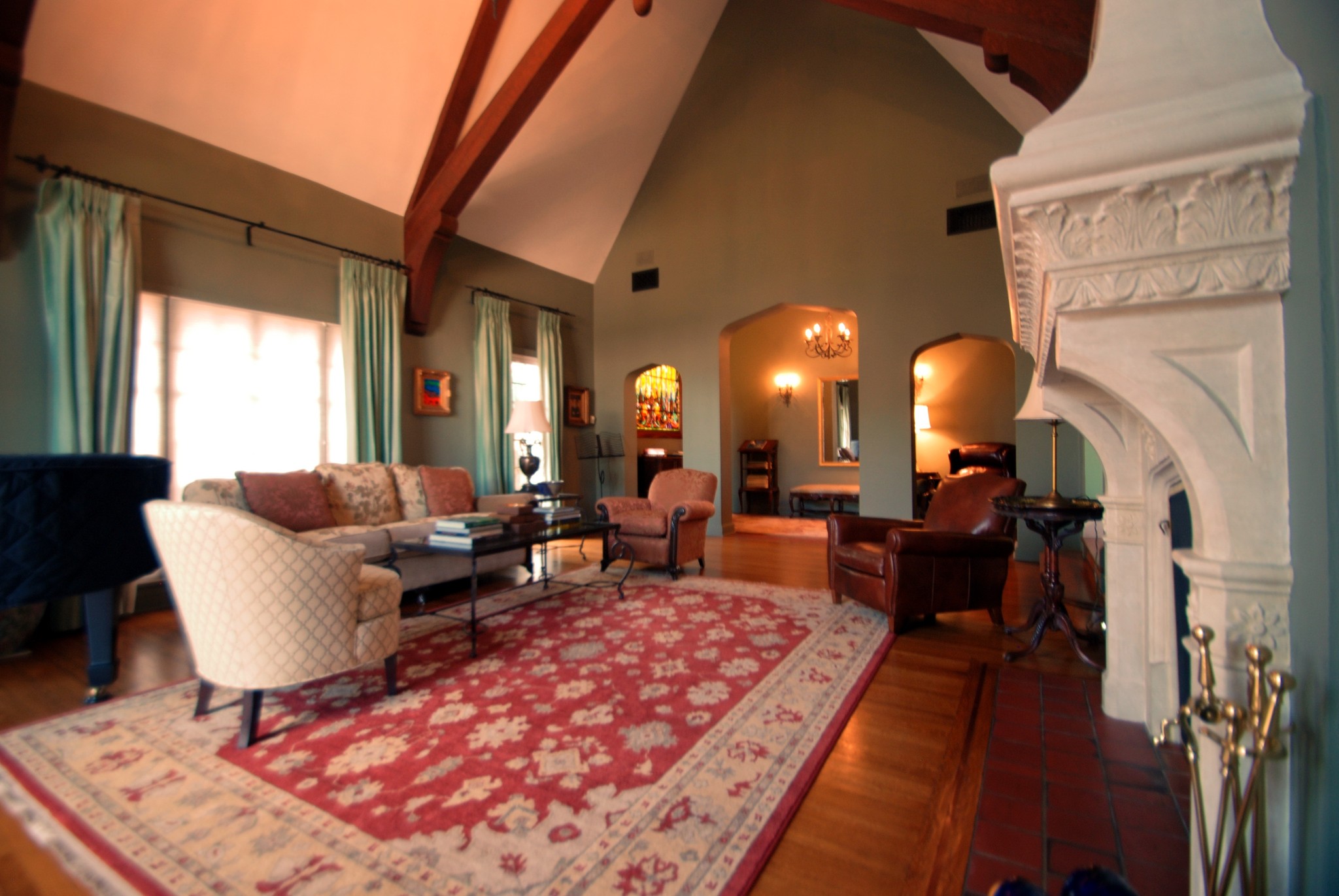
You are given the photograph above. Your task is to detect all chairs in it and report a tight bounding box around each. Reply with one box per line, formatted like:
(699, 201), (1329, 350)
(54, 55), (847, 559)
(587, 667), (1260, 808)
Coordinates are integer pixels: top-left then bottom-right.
(595, 469), (718, 580)
(141, 500), (403, 748)
(826, 442), (1026, 635)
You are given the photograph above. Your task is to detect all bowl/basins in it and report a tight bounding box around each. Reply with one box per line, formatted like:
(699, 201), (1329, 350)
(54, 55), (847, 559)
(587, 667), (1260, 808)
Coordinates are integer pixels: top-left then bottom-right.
(537, 480), (564, 496)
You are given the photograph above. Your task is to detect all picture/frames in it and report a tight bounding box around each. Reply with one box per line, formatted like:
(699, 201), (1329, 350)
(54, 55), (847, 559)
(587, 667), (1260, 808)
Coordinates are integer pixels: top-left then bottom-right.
(414, 367), (452, 416)
(565, 386), (589, 426)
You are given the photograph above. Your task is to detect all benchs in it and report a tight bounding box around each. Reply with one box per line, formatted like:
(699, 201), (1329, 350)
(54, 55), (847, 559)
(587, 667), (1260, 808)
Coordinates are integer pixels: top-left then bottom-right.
(788, 483), (860, 518)
(182, 479), (539, 595)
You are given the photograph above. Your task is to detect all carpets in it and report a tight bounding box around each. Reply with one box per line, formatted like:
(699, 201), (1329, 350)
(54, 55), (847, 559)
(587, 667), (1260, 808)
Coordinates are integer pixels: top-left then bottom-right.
(1, 560), (898, 896)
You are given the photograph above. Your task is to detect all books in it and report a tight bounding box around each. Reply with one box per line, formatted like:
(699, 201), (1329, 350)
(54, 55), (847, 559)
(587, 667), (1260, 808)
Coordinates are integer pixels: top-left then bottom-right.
(534, 507), (581, 526)
(429, 515), (504, 545)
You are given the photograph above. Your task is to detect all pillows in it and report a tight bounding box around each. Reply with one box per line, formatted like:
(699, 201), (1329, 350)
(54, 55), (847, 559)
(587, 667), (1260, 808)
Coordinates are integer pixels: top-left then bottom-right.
(235, 470), (338, 531)
(419, 466), (476, 516)
(839, 447), (855, 462)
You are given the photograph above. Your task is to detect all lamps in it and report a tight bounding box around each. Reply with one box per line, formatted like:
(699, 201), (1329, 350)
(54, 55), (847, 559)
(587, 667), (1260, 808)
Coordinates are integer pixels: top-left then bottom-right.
(504, 401), (553, 493)
(775, 372), (802, 405)
(1013, 366), (1070, 507)
(804, 312), (853, 359)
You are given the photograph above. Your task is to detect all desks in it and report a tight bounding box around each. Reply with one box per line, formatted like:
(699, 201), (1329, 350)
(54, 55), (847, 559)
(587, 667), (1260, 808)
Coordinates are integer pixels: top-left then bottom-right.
(383, 522), (635, 659)
(988, 496), (1105, 670)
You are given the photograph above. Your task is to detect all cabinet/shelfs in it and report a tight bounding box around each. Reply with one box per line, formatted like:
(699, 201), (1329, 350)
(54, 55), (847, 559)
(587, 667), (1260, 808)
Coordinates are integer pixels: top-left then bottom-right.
(535, 492), (590, 561)
(737, 440), (780, 516)
(637, 456), (682, 498)
